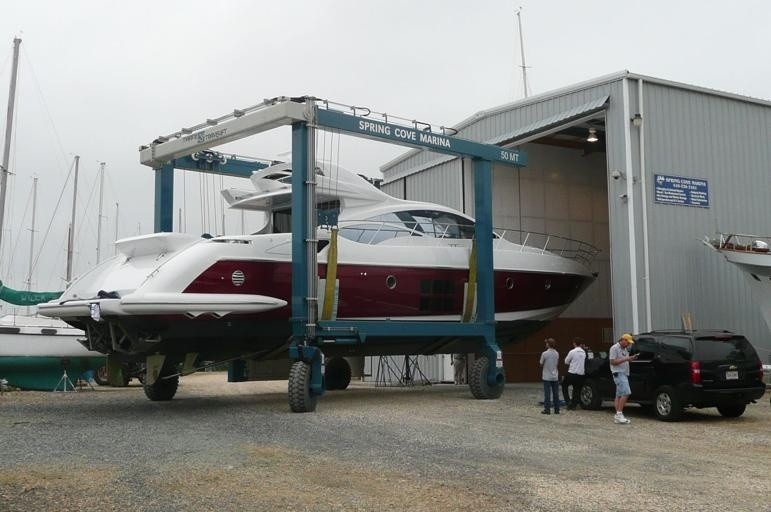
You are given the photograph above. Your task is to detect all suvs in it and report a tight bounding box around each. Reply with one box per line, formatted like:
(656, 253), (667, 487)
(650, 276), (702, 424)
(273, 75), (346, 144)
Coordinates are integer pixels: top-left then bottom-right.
(575, 329), (766, 423)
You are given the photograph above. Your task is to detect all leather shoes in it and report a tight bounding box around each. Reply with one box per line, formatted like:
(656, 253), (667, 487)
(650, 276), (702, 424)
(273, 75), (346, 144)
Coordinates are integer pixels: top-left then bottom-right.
(541, 402), (577, 414)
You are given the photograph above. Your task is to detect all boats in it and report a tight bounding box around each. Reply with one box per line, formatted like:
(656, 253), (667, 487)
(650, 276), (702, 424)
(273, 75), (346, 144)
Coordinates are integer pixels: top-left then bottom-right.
(698, 232), (771, 330)
(40, 154), (601, 359)
(0, 314), (111, 390)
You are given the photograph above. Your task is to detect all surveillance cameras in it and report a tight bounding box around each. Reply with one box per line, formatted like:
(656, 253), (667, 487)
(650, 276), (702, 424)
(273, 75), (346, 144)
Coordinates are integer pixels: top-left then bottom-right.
(613, 168), (627, 180)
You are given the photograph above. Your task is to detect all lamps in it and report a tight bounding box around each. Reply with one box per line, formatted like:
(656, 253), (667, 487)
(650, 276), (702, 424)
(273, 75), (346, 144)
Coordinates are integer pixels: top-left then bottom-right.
(585, 127), (600, 143)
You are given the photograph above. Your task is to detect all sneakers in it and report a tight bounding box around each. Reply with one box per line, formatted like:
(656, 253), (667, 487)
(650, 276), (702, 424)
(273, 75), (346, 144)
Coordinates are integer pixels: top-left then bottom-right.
(614, 414), (627, 423)
(614, 419), (630, 424)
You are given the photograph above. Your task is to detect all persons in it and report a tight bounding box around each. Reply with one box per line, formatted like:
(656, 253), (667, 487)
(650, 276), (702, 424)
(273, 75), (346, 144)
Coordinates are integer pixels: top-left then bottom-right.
(562, 337), (587, 409)
(579, 338), (595, 366)
(610, 333), (640, 425)
(539, 338), (561, 414)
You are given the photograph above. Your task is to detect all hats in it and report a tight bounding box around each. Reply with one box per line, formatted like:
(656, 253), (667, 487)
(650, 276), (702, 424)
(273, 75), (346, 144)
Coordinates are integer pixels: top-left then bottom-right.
(544, 338), (555, 346)
(621, 334), (634, 344)
